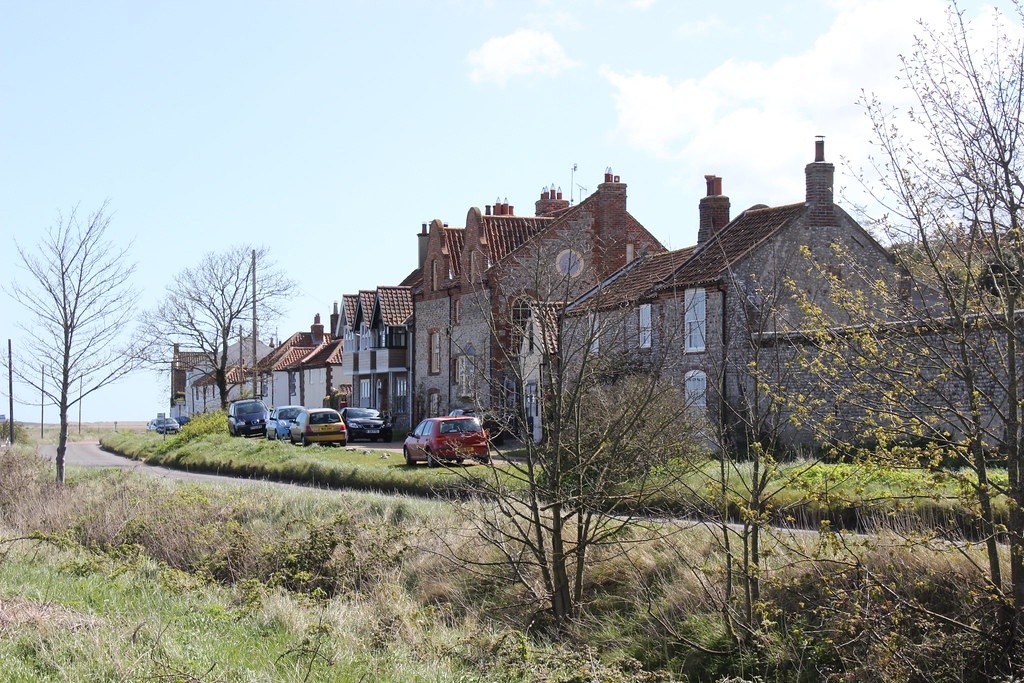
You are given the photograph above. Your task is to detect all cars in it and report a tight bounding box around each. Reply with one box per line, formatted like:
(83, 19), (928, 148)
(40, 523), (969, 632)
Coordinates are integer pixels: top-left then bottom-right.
(175, 416), (191, 426)
(403, 414), (490, 468)
(289, 409), (348, 447)
(266, 406), (305, 441)
(155, 419), (180, 434)
(147, 419), (156, 432)
(339, 407), (393, 443)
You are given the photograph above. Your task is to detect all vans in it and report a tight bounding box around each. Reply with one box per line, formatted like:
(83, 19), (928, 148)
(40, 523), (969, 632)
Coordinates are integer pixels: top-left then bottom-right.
(228, 399), (270, 438)
